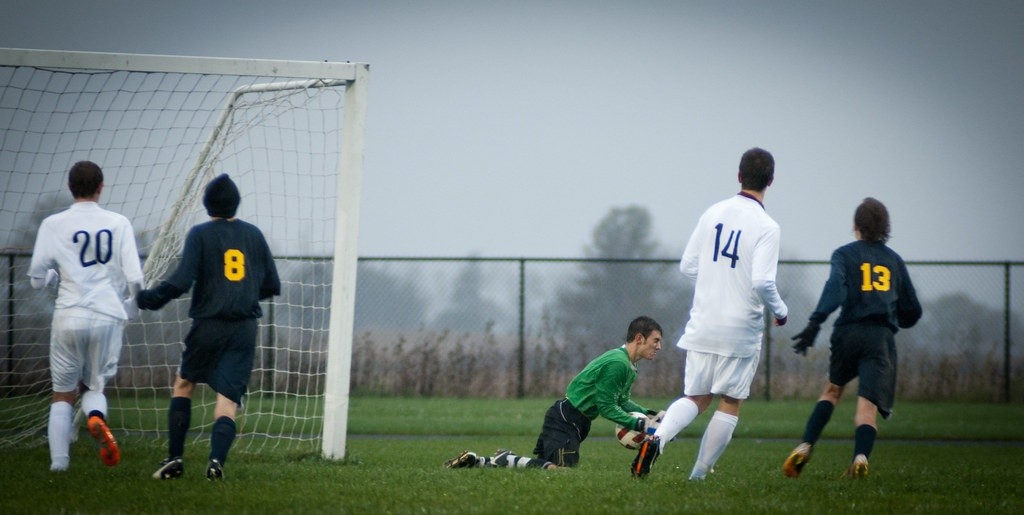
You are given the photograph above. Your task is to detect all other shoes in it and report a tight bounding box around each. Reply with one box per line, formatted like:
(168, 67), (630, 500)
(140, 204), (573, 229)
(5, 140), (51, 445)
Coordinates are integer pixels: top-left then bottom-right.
(853, 455), (868, 480)
(152, 457), (184, 481)
(206, 458), (223, 481)
(484, 448), (516, 469)
(630, 437), (660, 478)
(443, 451), (479, 469)
(784, 443), (811, 477)
(87, 414), (120, 466)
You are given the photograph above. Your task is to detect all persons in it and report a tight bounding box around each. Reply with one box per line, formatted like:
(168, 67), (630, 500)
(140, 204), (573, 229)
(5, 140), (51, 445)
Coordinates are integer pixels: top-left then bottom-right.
(138, 174), (283, 481)
(784, 197), (924, 481)
(446, 315), (663, 476)
(28, 161), (147, 469)
(627, 148), (791, 481)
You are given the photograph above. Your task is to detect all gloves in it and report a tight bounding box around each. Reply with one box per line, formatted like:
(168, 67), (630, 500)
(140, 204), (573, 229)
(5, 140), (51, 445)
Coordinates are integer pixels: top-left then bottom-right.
(791, 325), (819, 358)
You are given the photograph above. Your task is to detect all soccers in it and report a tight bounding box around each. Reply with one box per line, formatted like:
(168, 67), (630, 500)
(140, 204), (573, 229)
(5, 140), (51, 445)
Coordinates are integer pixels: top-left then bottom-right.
(615, 411), (651, 450)
